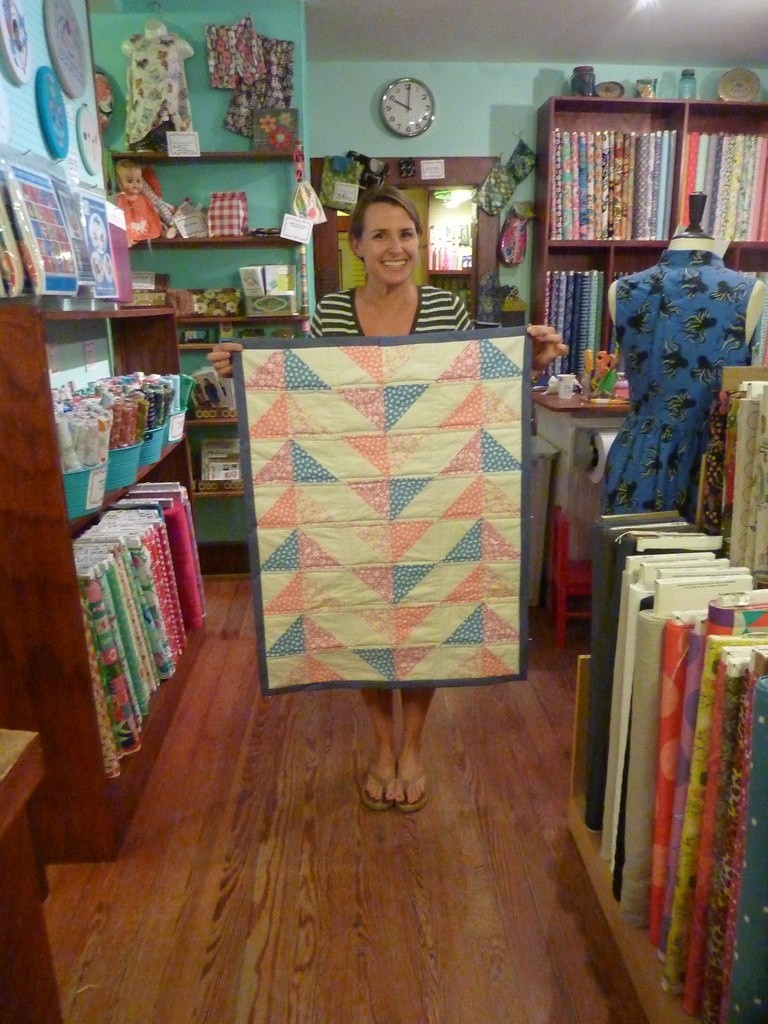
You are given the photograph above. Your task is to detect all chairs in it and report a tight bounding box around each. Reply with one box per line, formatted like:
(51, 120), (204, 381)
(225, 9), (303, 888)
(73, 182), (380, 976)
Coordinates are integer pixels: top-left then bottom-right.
(552, 504), (593, 644)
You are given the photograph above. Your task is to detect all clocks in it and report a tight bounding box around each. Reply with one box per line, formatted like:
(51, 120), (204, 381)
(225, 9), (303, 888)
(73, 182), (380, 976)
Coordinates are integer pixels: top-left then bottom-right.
(381, 78), (436, 138)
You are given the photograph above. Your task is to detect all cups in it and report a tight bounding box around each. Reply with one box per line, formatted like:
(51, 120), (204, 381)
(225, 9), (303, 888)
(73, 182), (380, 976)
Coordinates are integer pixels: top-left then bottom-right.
(557, 374), (576, 399)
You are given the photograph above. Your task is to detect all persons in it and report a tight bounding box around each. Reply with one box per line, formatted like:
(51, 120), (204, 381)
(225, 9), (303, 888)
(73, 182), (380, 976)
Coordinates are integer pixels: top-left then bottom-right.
(206, 187), (569, 812)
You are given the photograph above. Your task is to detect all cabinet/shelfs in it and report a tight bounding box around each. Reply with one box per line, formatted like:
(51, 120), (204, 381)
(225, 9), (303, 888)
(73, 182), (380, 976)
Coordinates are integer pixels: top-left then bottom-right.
(110, 151), (312, 495)
(0, 291), (209, 867)
(527, 96), (768, 434)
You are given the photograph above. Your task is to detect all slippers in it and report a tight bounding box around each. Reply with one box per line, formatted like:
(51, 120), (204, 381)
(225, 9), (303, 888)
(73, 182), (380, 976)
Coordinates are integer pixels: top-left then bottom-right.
(362, 764), (395, 811)
(395, 765), (429, 811)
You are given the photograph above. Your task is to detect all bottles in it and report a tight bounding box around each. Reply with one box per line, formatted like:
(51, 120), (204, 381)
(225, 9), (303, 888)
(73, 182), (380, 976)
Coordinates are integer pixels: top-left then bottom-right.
(677, 69), (697, 100)
